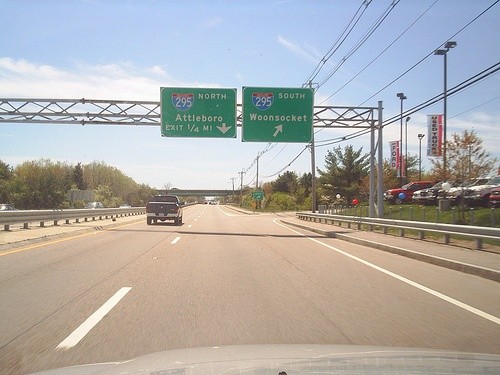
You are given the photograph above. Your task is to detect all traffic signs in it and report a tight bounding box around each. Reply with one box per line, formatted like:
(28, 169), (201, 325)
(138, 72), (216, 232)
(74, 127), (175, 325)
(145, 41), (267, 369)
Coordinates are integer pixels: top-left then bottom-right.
(159, 86), (238, 138)
(251, 191), (263, 200)
(240, 85), (315, 144)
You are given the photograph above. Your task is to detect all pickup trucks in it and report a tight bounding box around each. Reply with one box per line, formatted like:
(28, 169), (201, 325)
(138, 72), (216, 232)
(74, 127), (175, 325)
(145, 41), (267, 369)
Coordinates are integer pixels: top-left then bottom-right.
(145, 195), (186, 226)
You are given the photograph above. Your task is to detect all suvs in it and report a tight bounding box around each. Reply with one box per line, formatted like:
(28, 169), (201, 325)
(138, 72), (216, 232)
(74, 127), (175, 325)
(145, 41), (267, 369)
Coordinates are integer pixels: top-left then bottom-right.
(383, 180), (434, 205)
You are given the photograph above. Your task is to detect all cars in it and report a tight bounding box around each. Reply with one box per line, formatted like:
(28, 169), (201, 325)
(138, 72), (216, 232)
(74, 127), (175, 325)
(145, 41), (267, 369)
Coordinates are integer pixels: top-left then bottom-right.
(0, 203), (15, 211)
(118, 204), (132, 208)
(83, 201), (104, 209)
(412, 175), (500, 210)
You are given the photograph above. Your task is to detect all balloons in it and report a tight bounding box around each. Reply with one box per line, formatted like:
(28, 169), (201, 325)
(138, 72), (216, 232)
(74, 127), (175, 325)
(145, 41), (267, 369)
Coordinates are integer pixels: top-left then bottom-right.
(353, 199), (358, 204)
(336, 194), (340, 198)
(442, 182), (450, 190)
(399, 193), (405, 199)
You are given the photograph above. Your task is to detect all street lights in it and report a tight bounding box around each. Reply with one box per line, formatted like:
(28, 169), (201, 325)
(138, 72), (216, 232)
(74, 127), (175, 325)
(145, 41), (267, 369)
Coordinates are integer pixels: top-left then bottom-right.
(396, 92), (407, 188)
(405, 117), (410, 184)
(433, 41), (457, 199)
